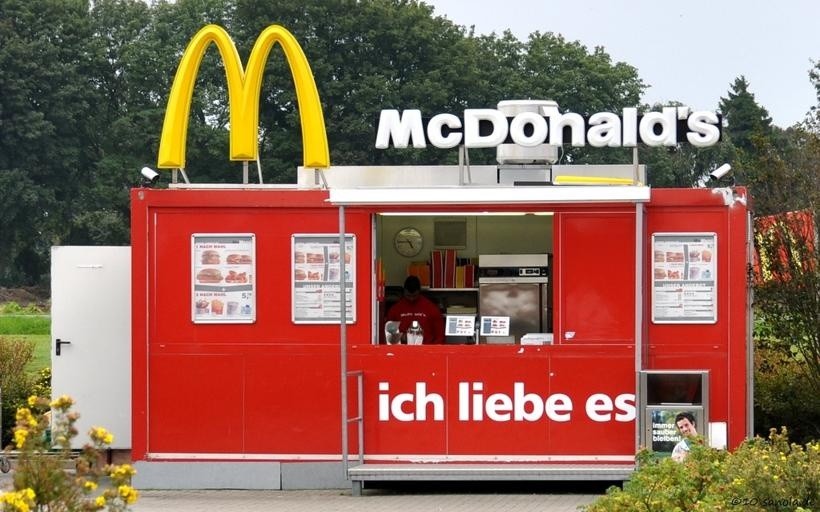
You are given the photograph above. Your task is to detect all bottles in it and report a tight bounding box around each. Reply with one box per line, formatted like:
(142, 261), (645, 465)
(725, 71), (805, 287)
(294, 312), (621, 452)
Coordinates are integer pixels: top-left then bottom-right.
(407, 320), (423, 345)
(705, 270), (712, 278)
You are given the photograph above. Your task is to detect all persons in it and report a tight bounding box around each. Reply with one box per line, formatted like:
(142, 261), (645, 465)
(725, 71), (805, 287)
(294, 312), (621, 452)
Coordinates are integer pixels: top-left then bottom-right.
(670, 410), (703, 464)
(384, 273), (446, 344)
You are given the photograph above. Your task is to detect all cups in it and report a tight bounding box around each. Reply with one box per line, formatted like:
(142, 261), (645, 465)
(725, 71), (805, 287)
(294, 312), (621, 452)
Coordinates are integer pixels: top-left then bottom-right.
(690, 268), (701, 279)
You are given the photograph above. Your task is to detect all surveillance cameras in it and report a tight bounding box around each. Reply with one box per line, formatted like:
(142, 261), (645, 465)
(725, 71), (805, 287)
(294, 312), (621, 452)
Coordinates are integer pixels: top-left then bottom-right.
(710, 163), (732, 181)
(141, 167), (160, 183)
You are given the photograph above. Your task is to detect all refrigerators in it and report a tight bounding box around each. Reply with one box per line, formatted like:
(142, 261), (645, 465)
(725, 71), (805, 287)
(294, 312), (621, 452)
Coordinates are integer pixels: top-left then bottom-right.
(477, 254), (551, 346)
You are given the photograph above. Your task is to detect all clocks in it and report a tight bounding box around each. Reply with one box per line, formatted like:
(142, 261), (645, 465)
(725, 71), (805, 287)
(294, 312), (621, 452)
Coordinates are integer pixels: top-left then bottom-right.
(392, 226), (424, 258)
